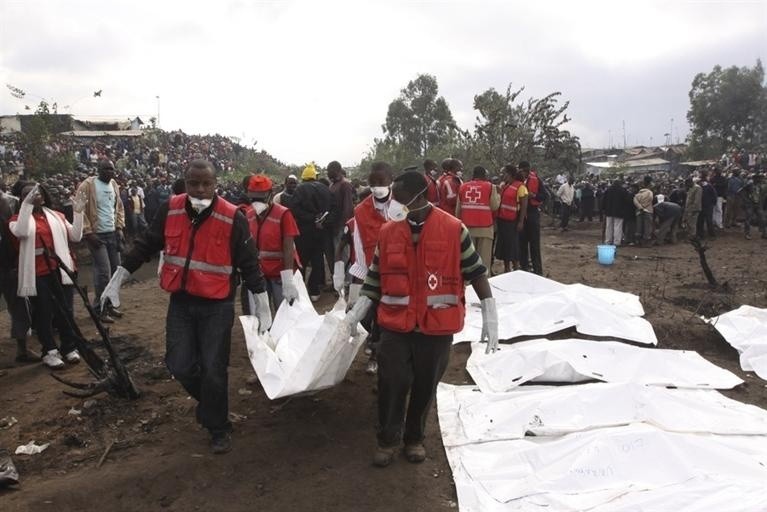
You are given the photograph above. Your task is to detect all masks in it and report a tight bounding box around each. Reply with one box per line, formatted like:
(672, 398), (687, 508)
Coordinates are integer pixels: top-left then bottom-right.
(250, 192), (273, 217)
(499, 172), (512, 190)
(455, 170), (463, 177)
(387, 185), (430, 222)
(369, 181), (394, 200)
(425, 166), (438, 177)
(185, 192), (216, 215)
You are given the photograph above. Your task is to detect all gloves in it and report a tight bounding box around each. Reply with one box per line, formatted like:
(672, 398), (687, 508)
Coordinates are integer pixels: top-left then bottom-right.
(98, 264), (133, 317)
(278, 269), (300, 307)
(332, 260), (345, 298)
(156, 249), (166, 280)
(343, 294), (373, 337)
(480, 297), (498, 355)
(346, 282), (364, 314)
(250, 290), (273, 334)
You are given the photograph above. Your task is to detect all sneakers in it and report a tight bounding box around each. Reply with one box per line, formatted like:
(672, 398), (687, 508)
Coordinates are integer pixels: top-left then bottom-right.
(209, 431), (233, 454)
(64, 349), (81, 365)
(15, 336), (41, 363)
(364, 343), (372, 355)
(40, 346), (66, 370)
(365, 358), (379, 375)
(245, 371), (257, 384)
(405, 442), (427, 463)
(372, 444), (400, 468)
(310, 293), (322, 302)
(194, 401), (204, 425)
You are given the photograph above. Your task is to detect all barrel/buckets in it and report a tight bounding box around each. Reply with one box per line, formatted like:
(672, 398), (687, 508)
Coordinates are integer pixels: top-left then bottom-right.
(597, 244), (616, 264)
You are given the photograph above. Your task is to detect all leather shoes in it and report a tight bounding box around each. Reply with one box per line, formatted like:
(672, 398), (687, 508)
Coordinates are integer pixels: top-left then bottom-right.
(109, 306), (125, 317)
(94, 310), (115, 323)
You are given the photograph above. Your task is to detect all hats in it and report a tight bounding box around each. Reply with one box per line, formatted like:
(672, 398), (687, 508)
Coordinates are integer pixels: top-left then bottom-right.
(247, 174), (273, 199)
(301, 163), (320, 180)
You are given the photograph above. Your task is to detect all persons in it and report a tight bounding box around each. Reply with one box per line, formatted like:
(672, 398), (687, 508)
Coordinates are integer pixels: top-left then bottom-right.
(317, 177), (334, 285)
(129, 161), (185, 177)
(295, 165), (338, 301)
(342, 172), (499, 467)
(489, 175), (502, 188)
(436, 158), (464, 217)
(151, 180), (185, 197)
(577, 173), (626, 181)
(89, 137), (185, 161)
(757, 187), (766, 237)
(697, 177), (717, 237)
(597, 184), (610, 190)
(7, 182), (88, 367)
(349, 162), (395, 372)
(726, 167), (754, 240)
(28, 172), (43, 182)
(355, 179), (369, 198)
(334, 188), (380, 376)
(272, 175), (306, 283)
(127, 187), (149, 234)
(218, 179), (240, 203)
(683, 166), (721, 176)
(187, 136), (232, 159)
(441, 160), (463, 215)
(128, 180), (146, 220)
(76, 159), (126, 322)
(602, 180), (627, 246)
(1, 133), (26, 178)
(547, 177), (556, 215)
(455, 167), (501, 277)
(741, 168), (766, 185)
(1, 189), (42, 364)
(655, 188), (681, 202)
(327, 162), (352, 295)
(241, 174), (300, 317)
(556, 176), (575, 232)
(216, 161), (236, 175)
(424, 159), (439, 206)
(579, 183), (594, 222)
(494, 165), (528, 273)
(711, 168), (725, 229)
(518, 162), (546, 274)
(44, 170), (74, 211)
(720, 145), (766, 167)
(627, 171), (681, 186)
(88, 149), (99, 176)
(490, 219), (499, 275)
(42, 134), (88, 158)
(681, 179), (703, 242)
(634, 188), (652, 244)
(556, 170), (566, 184)
(653, 202), (681, 245)
(596, 185), (605, 222)
(101, 160), (274, 454)
